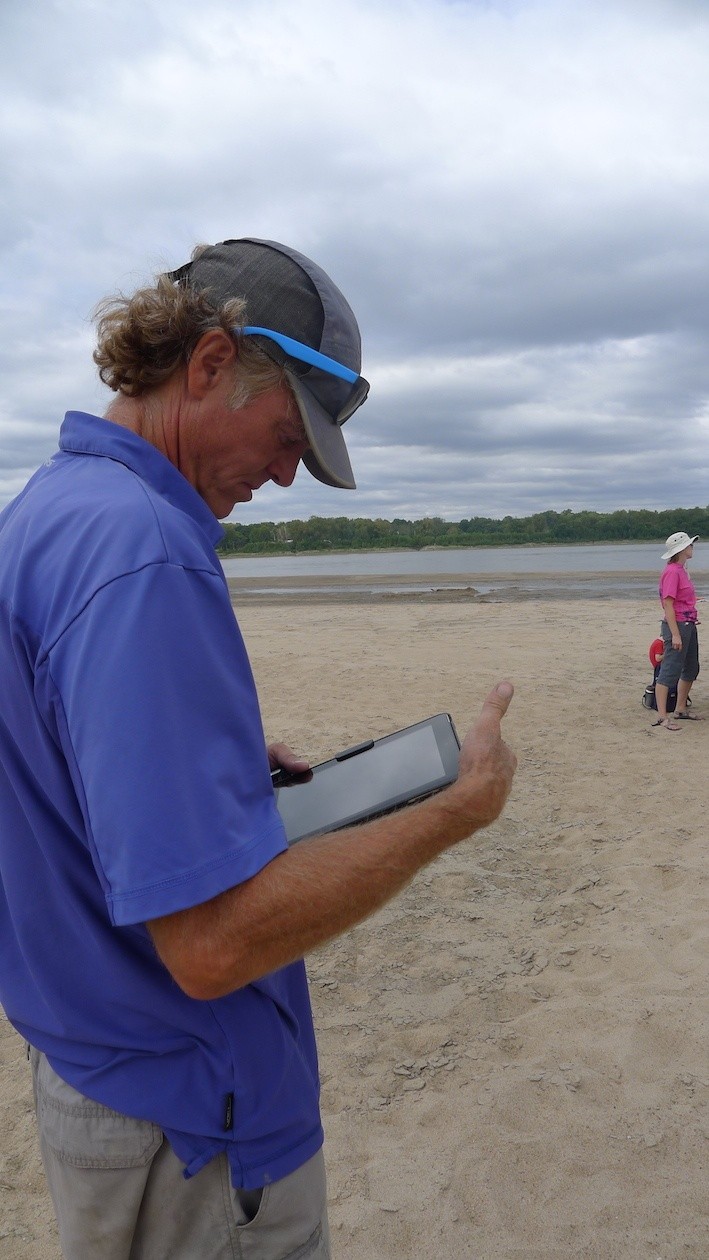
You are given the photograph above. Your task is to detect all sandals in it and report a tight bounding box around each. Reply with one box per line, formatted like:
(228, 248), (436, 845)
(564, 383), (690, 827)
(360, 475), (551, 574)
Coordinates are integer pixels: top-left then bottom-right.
(672, 709), (703, 721)
(658, 717), (682, 730)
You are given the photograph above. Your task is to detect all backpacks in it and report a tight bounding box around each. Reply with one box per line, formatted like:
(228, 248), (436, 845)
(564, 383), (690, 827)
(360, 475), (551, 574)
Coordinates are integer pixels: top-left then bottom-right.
(642, 662), (693, 713)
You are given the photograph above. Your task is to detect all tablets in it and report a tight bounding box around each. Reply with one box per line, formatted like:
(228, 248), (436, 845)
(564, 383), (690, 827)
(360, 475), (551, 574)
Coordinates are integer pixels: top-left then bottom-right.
(272, 711), (461, 848)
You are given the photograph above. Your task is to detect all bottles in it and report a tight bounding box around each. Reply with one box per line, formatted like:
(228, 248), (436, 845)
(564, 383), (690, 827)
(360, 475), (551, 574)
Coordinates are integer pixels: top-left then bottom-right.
(644, 686), (655, 706)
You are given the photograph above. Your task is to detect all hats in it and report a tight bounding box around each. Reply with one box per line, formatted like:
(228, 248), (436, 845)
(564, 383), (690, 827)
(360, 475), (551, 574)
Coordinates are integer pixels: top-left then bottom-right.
(660, 532), (700, 560)
(158, 235), (364, 490)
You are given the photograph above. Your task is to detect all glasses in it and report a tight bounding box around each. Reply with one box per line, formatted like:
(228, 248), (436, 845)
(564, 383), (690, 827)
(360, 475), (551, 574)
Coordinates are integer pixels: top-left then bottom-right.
(690, 543), (693, 547)
(229, 323), (371, 428)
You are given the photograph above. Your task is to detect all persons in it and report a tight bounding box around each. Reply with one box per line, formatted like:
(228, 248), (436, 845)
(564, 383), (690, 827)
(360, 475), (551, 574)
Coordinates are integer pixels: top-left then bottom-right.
(655, 531), (705, 731)
(0, 239), (520, 1260)
